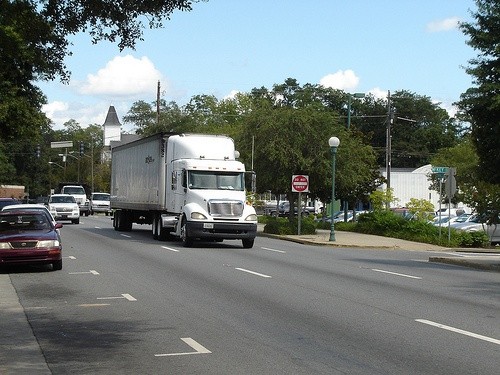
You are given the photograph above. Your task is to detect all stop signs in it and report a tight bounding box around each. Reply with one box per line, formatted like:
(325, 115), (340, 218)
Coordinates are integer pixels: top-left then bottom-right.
(292, 175), (310, 193)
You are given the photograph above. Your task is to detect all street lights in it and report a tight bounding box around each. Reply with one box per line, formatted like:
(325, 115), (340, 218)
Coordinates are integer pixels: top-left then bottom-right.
(48, 151), (94, 193)
(344, 92), (365, 224)
(328, 137), (340, 242)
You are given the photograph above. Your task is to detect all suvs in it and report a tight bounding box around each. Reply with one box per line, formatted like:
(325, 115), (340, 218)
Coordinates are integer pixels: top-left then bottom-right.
(48, 193), (81, 225)
(89, 192), (114, 217)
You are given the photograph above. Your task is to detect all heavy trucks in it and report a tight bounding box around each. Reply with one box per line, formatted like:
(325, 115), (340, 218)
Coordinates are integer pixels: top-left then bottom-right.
(109, 132), (258, 249)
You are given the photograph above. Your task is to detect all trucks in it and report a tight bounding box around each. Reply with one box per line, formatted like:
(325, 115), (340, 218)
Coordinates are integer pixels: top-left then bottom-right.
(56, 182), (90, 217)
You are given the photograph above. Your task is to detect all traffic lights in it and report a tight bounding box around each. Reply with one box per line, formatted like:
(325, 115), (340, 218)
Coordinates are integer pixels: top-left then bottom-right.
(79, 142), (84, 157)
(36, 144), (40, 158)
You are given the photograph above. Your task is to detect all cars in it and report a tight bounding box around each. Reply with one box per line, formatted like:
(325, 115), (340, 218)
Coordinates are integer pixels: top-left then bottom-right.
(0, 208), (64, 271)
(1, 204), (61, 236)
(0, 197), (22, 212)
(311, 207), (500, 246)
(249, 199), (315, 219)
